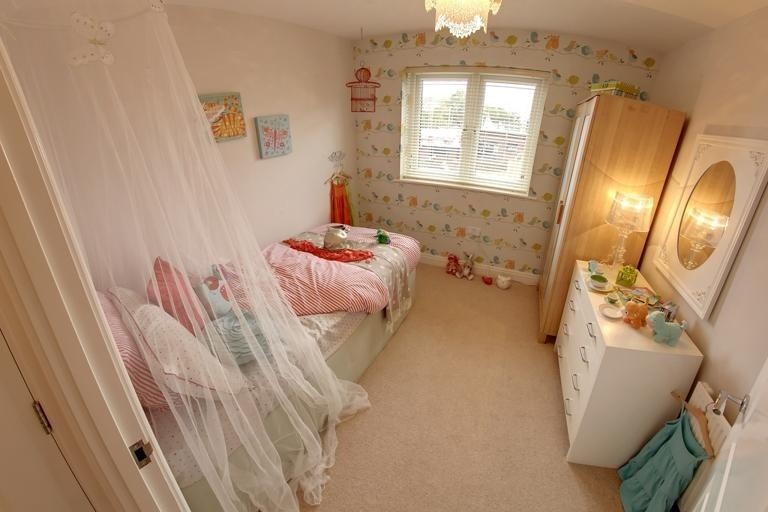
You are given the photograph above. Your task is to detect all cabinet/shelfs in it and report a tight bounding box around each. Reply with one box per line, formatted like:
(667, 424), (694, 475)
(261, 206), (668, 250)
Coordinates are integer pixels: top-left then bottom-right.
(536, 92), (687, 344)
(553, 256), (704, 468)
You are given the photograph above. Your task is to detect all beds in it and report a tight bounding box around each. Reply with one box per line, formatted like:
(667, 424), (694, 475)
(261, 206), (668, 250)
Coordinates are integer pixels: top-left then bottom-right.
(146, 221), (420, 512)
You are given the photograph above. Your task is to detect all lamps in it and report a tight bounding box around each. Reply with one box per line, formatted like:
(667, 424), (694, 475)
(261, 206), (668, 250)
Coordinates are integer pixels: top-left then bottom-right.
(424, 1), (502, 41)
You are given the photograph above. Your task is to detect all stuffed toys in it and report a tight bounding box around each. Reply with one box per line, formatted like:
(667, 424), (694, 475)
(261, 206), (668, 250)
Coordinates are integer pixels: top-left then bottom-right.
(198, 261), (234, 316)
(495, 273), (512, 290)
(481, 271), (493, 285)
(455, 254), (474, 281)
(373, 228), (391, 244)
(445, 254), (459, 275)
(323, 226), (353, 252)
(622, 294), (649, 330)
(645, 309), (688, 347)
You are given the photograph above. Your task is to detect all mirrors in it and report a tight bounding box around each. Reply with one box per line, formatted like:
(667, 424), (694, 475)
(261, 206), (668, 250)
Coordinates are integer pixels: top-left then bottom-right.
(652, 133), (768, 322)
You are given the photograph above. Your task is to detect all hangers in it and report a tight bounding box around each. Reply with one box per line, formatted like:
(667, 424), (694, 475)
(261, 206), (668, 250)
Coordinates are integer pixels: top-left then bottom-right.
(324, 163), (352, 184)
(669, 390), (723, 457)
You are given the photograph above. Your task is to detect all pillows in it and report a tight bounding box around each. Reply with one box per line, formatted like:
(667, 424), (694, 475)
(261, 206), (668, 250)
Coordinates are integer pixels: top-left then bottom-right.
(107, 286), (254, 402)
(197, 303), (293, 367)
(95, 289), (194, 413)
(148, 256), (208, 334)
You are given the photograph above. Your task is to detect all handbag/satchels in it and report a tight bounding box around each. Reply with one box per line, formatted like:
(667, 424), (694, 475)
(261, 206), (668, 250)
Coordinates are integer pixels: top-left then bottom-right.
(324, 224), (350, 249)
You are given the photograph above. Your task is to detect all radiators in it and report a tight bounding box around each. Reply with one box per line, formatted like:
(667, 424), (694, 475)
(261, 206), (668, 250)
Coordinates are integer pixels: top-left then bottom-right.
(676, 382), (732, 512)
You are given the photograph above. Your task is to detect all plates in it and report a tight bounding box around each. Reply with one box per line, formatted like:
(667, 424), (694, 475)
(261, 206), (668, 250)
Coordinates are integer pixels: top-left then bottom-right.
(599, 303), (623, 319)
(587, 277), (613, 291)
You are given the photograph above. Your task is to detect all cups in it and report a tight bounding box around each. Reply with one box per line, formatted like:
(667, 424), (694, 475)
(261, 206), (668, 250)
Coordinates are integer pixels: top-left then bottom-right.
(589, 275), (609, 288)
(604, 293), (619, 304)
